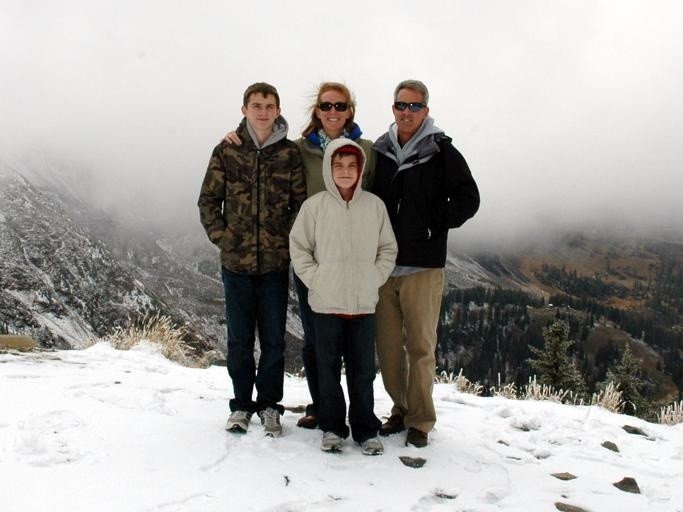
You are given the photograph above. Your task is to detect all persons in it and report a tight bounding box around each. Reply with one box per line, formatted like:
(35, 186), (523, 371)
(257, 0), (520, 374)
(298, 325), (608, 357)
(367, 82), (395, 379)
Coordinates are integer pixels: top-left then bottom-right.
(363, 79), (480, 450)
(196, 83), (305, 436)
(221, 81), (382, 431)
(286, 137), (400, 454)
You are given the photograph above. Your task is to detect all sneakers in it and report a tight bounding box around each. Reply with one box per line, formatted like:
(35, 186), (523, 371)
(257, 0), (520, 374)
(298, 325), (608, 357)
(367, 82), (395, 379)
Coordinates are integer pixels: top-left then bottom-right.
(321, 431), (344, 452)
(353, 438), (383, 455)
(259, 407), (282, 437)
(225, 410), (252, 433)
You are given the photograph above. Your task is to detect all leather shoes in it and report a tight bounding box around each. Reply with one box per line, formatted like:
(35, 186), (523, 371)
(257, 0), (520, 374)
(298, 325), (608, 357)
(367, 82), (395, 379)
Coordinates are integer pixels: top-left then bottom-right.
(297, 416), (318, 429)
(379, 414), (406, 436)
(406, 427), (427, 447)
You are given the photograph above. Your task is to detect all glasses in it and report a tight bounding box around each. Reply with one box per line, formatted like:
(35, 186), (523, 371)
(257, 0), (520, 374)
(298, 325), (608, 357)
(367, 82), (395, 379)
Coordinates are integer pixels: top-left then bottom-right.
(318, 102), (351, 111)
(394, 101), (426, 112)
